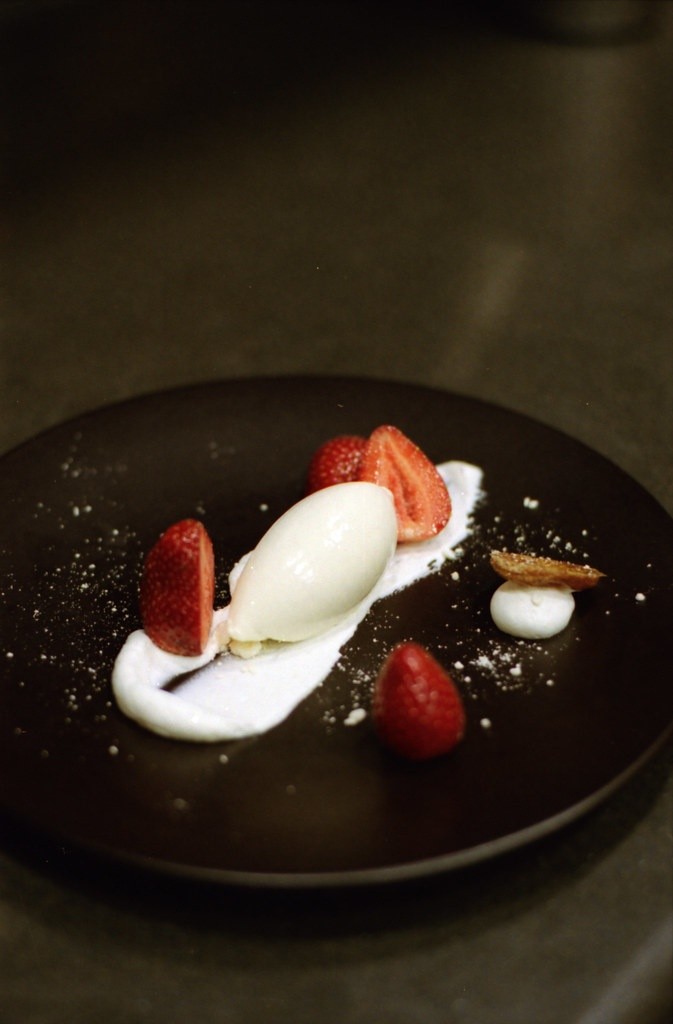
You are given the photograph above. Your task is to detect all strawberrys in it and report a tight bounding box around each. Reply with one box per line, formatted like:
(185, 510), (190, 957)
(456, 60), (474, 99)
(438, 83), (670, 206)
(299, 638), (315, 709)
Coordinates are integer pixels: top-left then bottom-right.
(371, 641), (464, 762)
(143, 517), (216, 655)
(307, 425), (453, 544)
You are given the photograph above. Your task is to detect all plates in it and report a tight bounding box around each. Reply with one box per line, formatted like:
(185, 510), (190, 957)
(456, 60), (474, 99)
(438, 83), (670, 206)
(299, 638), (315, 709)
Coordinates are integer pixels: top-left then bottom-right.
(2, 374), (670, 930)
